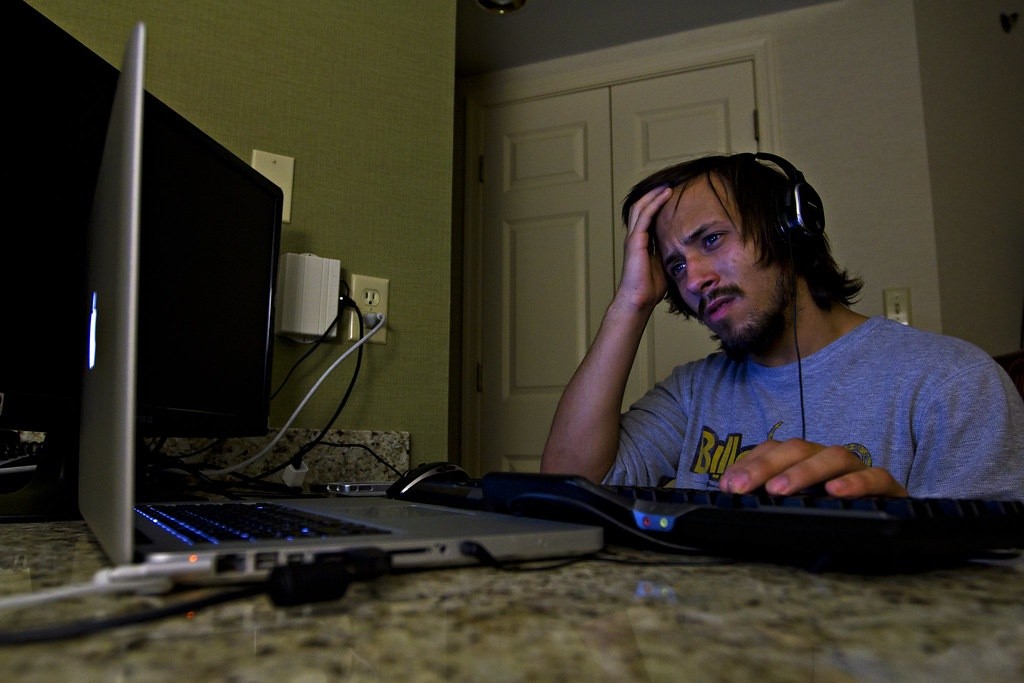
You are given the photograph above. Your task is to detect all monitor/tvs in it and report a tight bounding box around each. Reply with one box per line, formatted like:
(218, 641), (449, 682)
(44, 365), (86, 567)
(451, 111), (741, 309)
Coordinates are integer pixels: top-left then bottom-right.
(0, 0), (284, 524)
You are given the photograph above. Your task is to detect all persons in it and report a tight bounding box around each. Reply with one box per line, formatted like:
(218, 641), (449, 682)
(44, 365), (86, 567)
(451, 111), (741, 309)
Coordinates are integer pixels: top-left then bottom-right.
(539, 150), (1023, 505)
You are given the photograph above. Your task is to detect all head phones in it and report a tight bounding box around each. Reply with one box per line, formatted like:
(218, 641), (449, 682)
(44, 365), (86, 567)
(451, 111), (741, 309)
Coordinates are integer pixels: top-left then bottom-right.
(727, 152), (825, 254)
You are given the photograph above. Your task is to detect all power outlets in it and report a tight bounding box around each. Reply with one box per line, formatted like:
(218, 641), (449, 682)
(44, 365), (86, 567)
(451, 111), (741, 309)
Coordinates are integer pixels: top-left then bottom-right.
(348, 273), (389, 343)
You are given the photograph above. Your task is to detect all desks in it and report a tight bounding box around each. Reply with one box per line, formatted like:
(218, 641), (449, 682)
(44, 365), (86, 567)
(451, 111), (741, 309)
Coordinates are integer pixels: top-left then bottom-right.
(0, 518), (1024, 683)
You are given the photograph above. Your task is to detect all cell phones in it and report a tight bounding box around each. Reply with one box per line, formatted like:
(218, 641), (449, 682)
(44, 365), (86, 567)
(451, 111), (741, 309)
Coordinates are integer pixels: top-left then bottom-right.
(309, 482), (396, 497)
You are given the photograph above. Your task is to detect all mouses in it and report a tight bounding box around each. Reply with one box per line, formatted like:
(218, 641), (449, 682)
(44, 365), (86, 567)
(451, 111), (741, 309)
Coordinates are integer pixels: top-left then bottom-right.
(390, 462), (472, 501)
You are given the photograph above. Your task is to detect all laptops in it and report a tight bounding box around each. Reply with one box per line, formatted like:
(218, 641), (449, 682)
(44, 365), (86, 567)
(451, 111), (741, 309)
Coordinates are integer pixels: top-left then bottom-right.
(78, 23), (604, 589)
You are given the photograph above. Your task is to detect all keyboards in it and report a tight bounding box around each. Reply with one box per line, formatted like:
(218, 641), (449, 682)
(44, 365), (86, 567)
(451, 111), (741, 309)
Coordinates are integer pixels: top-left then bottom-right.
(421, 472), (1024, 570)
